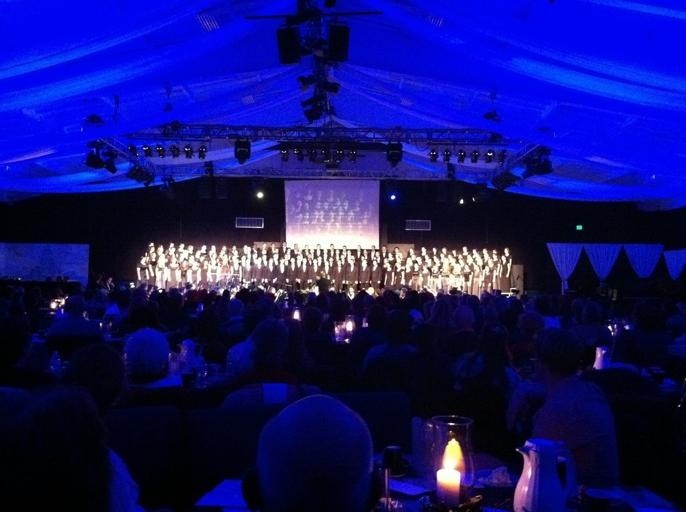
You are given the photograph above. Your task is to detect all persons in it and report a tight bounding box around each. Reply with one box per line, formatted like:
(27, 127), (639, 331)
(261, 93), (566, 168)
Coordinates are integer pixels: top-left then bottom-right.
(0, 242), (686, 512)
(286, 180), (377, 245)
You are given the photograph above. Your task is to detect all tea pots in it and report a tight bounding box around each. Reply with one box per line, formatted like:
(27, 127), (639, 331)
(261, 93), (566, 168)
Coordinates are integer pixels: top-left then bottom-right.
(512, 437), (575, 512)
(593, 346), (609, 370)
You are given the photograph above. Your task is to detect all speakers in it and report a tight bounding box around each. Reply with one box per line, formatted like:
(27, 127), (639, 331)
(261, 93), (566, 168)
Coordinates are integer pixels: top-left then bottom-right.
(510, 265), (524, 295)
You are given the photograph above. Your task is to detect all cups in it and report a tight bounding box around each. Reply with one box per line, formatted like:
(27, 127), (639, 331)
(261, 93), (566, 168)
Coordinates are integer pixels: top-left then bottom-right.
(422, 415), (476, 509)
(380, 439), (414, 477)
